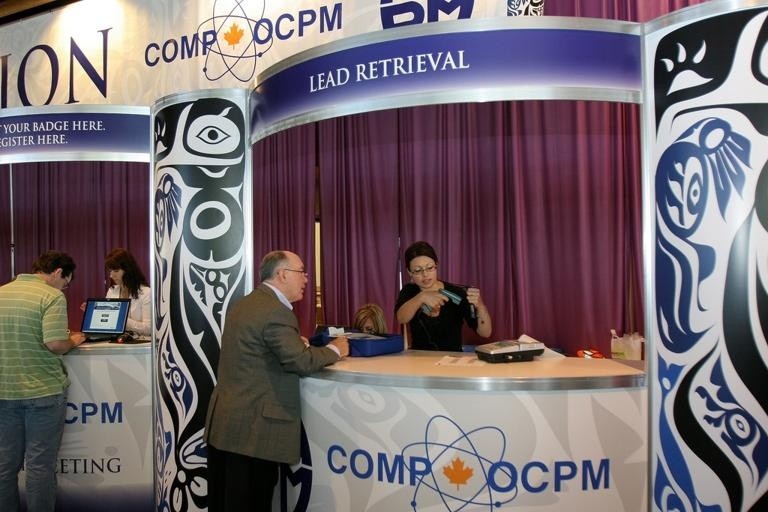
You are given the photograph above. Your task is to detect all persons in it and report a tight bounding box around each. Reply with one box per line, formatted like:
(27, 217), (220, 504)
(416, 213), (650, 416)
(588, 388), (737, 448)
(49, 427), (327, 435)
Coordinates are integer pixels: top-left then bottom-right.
(352, 303), (388, 336)
(0, 250), (87, 512)
(203, 251), (349, 512)
(79, 248), (151, 336)
(393, 240), (492, 353)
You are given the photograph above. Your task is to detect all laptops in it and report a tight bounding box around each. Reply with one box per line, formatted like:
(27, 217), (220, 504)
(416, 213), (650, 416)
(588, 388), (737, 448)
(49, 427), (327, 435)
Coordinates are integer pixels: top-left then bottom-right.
(80, 298), (131, 341)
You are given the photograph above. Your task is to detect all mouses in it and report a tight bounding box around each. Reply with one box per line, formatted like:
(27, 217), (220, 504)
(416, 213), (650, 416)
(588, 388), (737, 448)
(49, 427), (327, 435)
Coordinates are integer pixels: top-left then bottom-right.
(117, 334), (132, 343)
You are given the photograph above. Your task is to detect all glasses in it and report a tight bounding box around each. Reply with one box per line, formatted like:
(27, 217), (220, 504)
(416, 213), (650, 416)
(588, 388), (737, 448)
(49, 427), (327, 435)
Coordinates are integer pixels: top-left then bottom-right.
(409, 262), (439, 276)
